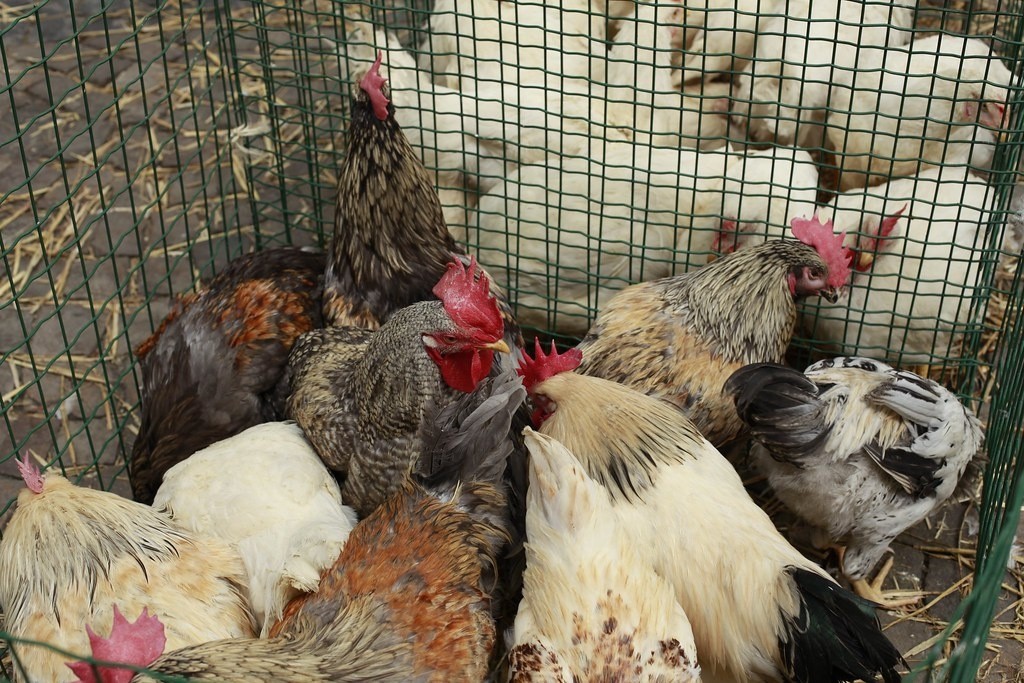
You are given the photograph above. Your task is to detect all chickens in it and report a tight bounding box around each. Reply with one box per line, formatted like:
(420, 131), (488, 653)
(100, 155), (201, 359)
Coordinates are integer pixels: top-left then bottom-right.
(3, 1), (1020, 681)
